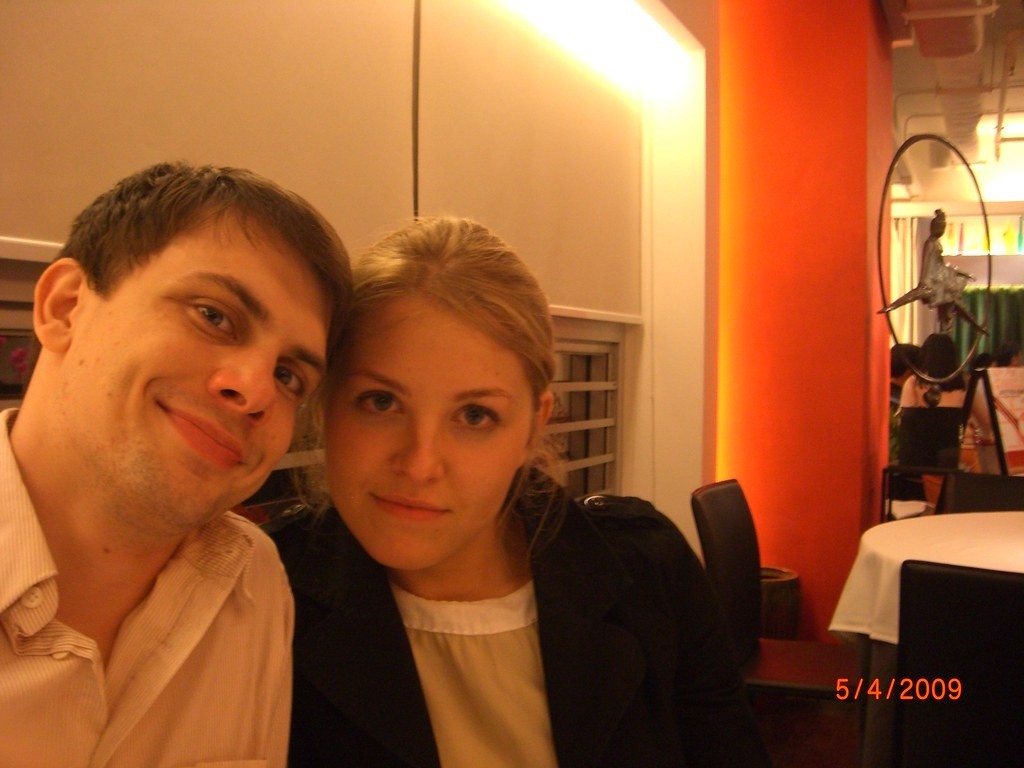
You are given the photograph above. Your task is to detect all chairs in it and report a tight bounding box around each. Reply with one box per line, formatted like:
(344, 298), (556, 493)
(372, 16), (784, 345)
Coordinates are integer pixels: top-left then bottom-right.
(935, 473), (1024, 515)
(889, 559), (1024, 768)
(691, 479), (865, 705)
(897, 407), (963, 468)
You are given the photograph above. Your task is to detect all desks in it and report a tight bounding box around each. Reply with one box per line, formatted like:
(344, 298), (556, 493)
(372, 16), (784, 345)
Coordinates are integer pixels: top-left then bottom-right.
(827, 507), (1024, 767)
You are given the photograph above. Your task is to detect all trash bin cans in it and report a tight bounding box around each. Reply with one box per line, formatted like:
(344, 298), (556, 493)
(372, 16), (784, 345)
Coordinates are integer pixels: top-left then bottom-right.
(760, 566), (799, 639)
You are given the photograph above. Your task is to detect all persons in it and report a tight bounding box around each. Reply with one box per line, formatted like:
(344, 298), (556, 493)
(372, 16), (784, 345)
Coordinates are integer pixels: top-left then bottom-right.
(0, 162), (353, 768)
(889, 333), (1023, 513)
(265, 216), (774, 768)
